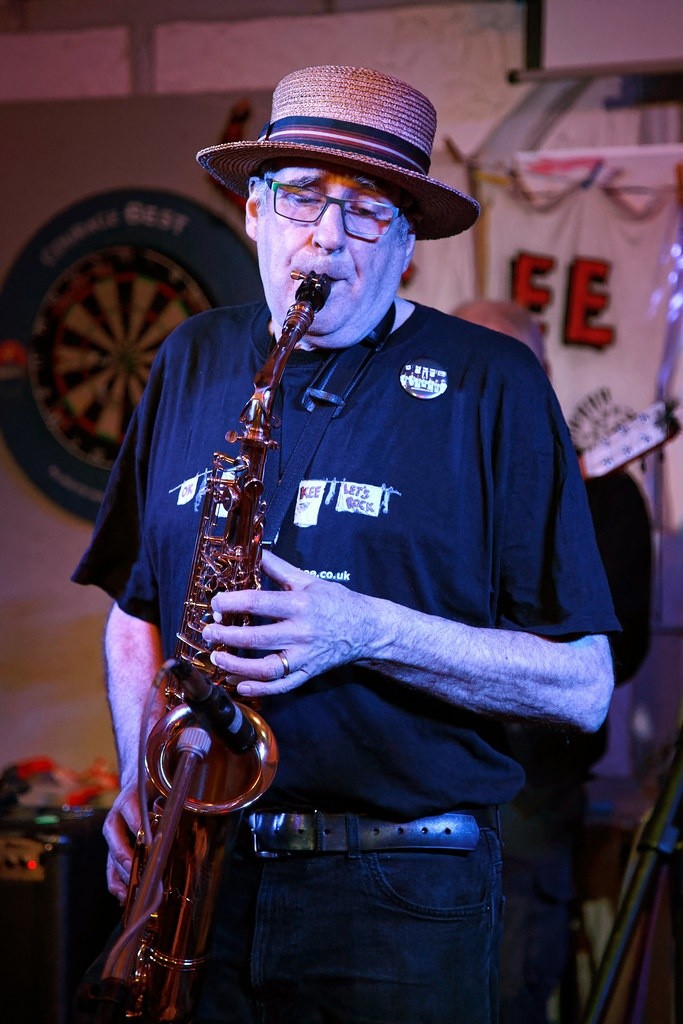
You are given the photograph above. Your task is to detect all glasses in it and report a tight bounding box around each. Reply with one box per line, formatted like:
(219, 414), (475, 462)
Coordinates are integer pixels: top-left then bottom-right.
(264, 175), (415, 243)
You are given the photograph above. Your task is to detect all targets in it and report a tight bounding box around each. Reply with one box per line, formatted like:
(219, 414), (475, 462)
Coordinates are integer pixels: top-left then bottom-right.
(32, 243), (215, 474)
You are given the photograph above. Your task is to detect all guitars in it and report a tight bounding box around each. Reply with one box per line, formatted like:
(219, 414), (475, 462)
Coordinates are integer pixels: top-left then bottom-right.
(578, 396), (683, 483)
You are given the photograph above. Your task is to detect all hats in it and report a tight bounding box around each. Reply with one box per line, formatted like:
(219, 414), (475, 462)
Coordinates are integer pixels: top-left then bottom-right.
(195, 65), (481, 241)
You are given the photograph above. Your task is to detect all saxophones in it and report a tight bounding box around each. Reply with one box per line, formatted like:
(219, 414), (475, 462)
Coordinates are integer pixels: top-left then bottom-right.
(102, 268), (335, 1024)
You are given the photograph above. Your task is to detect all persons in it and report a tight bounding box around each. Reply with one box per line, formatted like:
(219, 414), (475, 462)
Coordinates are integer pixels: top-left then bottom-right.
(439, 301), (655, 1023)
(69, 64), (625, 1024)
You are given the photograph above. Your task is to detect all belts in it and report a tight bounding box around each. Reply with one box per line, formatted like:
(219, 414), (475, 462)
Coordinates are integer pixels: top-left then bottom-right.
(242, 803), (499, 861)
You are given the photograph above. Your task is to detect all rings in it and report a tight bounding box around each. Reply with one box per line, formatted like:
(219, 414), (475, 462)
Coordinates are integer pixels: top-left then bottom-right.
(277, 652), (289, 678)
